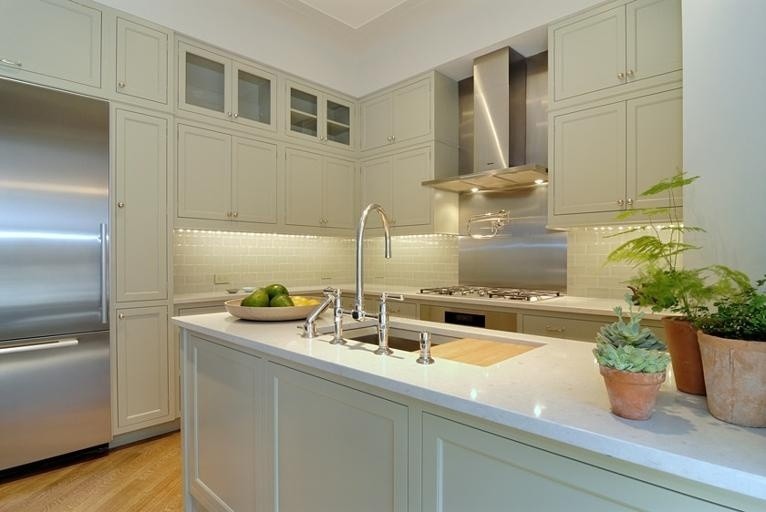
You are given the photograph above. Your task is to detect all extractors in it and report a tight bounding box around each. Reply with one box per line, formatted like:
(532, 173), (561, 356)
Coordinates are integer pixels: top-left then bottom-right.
(419, 46), (548, 194)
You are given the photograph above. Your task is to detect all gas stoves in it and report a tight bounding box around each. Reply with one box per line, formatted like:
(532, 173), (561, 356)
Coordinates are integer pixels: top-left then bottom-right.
(418, 283), (566, 304)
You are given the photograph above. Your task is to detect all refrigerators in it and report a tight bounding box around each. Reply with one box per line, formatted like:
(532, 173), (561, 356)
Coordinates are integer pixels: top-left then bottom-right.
(0, 75), (112, 479)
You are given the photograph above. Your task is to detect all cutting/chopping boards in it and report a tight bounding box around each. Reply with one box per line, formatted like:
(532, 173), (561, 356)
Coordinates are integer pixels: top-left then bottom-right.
(411, 337), (535, 368)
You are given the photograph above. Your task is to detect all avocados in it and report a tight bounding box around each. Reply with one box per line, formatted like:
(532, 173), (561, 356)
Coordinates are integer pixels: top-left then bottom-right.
(265, 284), (289, 301)
(270, 294), (294, 307)
(240, 288), (269, 306)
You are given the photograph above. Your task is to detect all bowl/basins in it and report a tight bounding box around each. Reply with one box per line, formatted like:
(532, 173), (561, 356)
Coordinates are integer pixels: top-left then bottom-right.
(225, 288), (240, 294)
(242, 287), (254, 293)
(223, 295), (326, 321)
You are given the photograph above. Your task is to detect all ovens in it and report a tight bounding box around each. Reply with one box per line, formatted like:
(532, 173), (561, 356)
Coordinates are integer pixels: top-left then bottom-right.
(419, 300), (517, 334)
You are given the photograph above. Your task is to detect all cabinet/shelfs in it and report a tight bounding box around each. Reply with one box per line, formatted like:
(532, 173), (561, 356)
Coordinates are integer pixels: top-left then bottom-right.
(0, 0), (108, 100)
(518, 308), (668, 353)
(173, 32), (281, 234)
(109, 5), (174, 115)
(110, 100), (173, 306)
(281, 71), (358, 237)
(358, 70), (459, 235)
(109, 306), (180, 449)
(547, 0), (682, 232)
(341, 295), (419, 325)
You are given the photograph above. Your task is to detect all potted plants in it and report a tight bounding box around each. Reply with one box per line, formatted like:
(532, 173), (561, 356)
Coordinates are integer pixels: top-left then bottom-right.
(693, 274), (766, 428)
(600, 170), (750, 395)
(593, 294), (669, 420)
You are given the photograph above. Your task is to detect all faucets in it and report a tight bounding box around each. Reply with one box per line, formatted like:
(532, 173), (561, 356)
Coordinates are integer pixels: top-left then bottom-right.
(355, 203), (391, 311)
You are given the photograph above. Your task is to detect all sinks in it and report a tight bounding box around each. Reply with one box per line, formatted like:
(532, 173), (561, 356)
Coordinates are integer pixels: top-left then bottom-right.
(315, 319), (464, 352)
